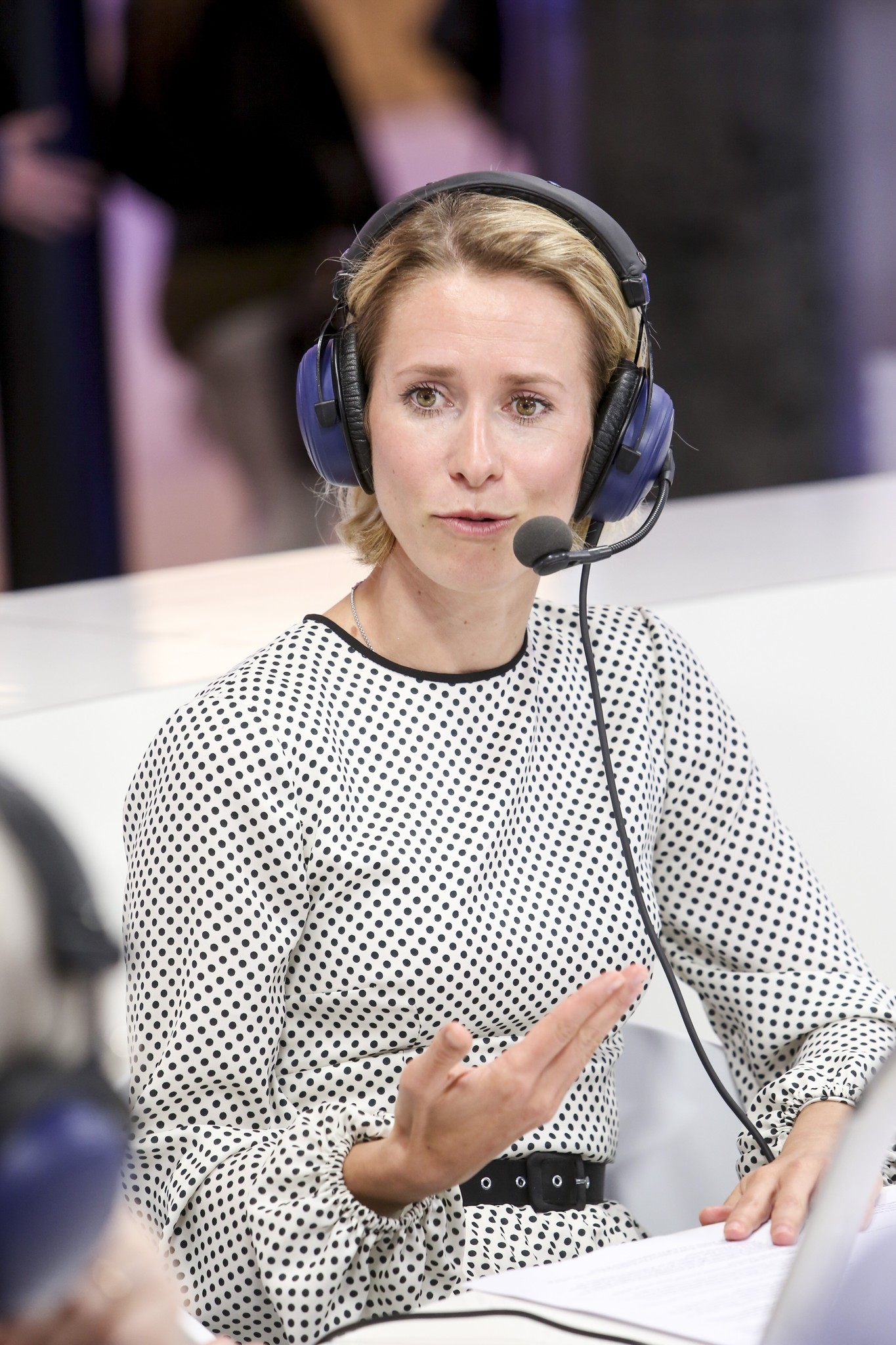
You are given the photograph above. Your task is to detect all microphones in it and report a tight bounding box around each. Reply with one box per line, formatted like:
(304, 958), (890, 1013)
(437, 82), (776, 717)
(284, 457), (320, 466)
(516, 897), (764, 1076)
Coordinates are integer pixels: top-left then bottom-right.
(512, 447), (677, 575)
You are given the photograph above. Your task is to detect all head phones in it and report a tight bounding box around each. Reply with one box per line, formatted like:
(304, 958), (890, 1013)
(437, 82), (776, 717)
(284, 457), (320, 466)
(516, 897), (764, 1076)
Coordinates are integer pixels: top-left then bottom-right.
(294, 170), (686, 520)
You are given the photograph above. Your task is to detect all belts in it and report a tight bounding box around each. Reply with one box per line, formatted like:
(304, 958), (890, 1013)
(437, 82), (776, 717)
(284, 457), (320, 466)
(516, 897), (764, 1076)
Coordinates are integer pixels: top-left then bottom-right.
(458, 1151), (604, 1211)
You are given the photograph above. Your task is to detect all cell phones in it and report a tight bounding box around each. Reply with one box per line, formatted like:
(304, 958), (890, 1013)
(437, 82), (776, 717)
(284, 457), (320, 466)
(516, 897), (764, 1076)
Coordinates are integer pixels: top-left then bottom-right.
(1, 1099), (129, 1316)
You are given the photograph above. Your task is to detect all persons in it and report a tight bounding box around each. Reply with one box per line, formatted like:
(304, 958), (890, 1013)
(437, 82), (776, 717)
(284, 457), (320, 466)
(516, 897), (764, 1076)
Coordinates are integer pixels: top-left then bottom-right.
(121, 193), (895, 1345)
(1, 0), (602, 599)
